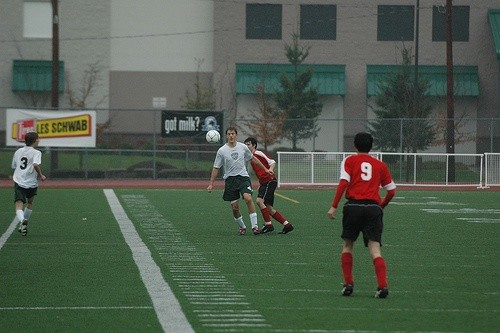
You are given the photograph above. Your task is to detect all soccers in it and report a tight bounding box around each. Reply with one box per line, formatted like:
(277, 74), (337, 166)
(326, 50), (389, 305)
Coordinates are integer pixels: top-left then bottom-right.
(206, 129), (220, 143)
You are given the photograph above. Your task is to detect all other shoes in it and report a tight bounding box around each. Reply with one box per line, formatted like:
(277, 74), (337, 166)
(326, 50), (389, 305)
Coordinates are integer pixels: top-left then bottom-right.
(278, 224), (294, 234)
(340, 284), (353, 296)
(239, 227), (246, 235)
(260, 224), (274, 234)
(252, 227), (259, 234)
(17, 219), (28, 236)
(373, 287), (390, 299)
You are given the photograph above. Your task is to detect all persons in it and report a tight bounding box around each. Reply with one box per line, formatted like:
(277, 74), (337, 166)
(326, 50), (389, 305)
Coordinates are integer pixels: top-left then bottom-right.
(244, 137), (294, 235)
(12, 132), (46, 236)
(206, 127), (275, 235)
(327, 132), (397, 298)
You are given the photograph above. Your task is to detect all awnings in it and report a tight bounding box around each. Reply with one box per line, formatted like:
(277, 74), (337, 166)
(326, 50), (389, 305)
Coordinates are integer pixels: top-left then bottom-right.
(488, 9), (500, 54)
(236, 63), (345, 96)
(366, 64), (480, 98)
(11, 59), (65, 94)
